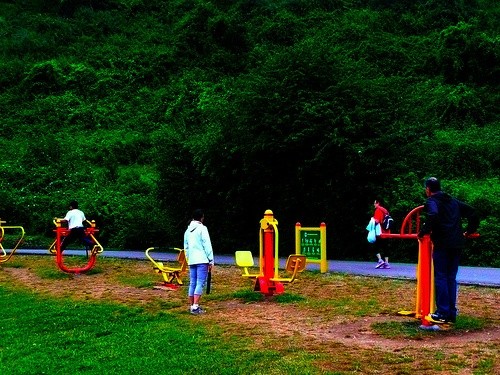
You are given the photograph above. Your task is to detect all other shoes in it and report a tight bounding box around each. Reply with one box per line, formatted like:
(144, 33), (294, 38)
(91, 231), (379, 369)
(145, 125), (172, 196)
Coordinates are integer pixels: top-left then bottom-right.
(447, 313), (457, 325)
(431, 313), (448, 322)
(380, 264), (390, 268)
(376, 261), (384, 268)
(191, 307), (206, 315)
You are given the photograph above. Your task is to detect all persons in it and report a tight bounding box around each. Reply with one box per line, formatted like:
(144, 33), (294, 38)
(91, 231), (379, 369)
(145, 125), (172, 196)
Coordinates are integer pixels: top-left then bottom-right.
(417, 177), (481, 323)
(183, 209), (215, 316)
(54, 202), (98, 254)
(370, 199), (390, 269)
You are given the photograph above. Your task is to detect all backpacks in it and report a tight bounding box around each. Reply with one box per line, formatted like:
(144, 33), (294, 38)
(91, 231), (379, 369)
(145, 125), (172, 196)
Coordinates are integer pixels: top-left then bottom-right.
(380, 213), (394, 229)
